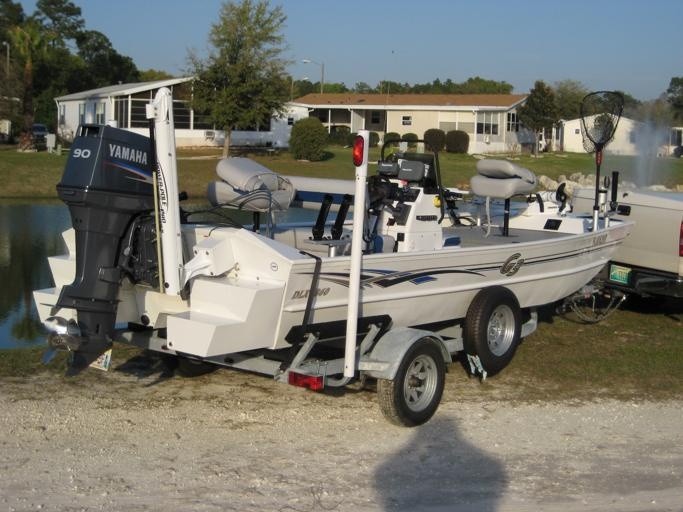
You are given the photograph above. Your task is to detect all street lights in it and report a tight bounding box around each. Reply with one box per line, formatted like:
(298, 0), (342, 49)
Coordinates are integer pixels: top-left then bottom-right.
(291, 78), (308, 101)
(2, 42), (10, 78)
(301, 60), (324, 94)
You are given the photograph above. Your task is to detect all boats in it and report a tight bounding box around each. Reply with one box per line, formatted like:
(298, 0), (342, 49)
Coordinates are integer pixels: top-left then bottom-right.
(32, 87), (635, 426)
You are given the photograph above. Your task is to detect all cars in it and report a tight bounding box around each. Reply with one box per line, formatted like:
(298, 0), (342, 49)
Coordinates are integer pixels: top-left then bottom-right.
(33, 123), (48, 140)
